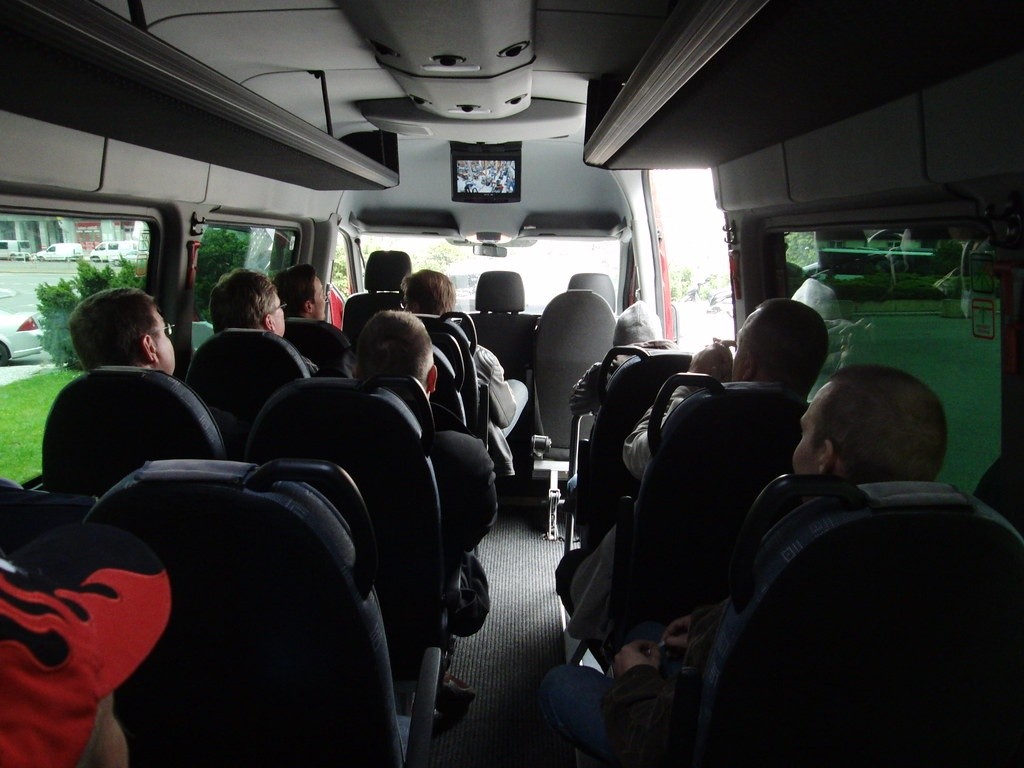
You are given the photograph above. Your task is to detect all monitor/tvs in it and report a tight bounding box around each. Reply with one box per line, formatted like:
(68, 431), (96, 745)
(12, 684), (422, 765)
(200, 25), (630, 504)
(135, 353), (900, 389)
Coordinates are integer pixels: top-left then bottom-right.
(454, 152), (521, 203)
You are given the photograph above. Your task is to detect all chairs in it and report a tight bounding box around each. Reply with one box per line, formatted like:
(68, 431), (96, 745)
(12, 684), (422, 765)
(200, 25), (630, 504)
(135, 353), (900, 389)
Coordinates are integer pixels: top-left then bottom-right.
(41, 251), (1024, 768)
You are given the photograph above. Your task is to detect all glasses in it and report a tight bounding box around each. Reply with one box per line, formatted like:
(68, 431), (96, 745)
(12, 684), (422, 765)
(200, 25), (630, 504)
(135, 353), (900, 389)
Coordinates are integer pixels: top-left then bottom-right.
(312, 289), (330, 307)
(399, 299), (409, 310)
(266, 301), (288, 319)
(148, 322), (175, 336)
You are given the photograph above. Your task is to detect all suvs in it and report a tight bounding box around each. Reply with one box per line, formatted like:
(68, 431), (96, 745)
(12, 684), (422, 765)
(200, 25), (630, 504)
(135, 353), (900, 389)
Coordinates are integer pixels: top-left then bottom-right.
(37, 243), (83, 263)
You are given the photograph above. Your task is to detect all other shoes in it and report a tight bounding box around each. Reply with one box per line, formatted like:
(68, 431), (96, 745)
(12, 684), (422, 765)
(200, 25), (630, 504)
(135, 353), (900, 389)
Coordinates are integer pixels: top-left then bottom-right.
(438, 672), (476, 710)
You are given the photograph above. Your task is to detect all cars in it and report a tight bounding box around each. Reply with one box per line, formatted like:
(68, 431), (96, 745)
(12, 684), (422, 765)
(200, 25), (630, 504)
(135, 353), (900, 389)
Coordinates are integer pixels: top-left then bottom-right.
(0, 311), (47, 367)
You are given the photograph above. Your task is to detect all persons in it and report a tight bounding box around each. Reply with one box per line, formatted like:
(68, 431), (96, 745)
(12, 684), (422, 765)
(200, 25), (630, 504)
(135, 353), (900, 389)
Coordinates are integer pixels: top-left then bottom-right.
(537, 363), (948, 768)
(273, 264), (329, 320)
(568, 301), (663, 513)
(782, 228), (985, 404)
(0, 523), (172, 768)
(210, 268), (319, 377)
(399, 269), (528, 478)
(567, 299), (829, 641)
(352, 310), (498, 705)
(69, 288), (247, 461)
(457, 161), (515, 193)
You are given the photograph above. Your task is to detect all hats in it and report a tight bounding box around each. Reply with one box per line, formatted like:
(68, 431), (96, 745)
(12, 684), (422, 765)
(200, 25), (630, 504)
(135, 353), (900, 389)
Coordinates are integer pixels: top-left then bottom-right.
(0, 558), (172, 768)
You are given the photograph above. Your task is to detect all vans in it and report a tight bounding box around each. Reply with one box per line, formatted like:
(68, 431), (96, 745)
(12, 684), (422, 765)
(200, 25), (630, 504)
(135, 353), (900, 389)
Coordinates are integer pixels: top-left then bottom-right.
(89, 240), (150, 263)
(0, 240), (36, 260)
